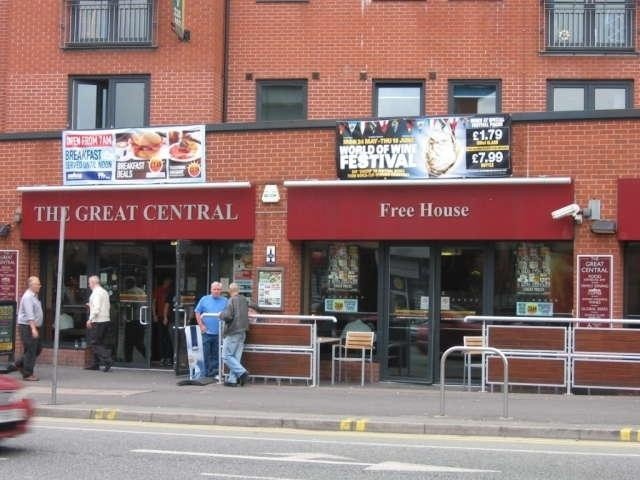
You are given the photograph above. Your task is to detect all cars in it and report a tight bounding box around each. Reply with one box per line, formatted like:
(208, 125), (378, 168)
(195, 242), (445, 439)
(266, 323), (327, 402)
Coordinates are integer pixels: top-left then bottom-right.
(406, 313), (485, 358)
(0, 370), (38, 440)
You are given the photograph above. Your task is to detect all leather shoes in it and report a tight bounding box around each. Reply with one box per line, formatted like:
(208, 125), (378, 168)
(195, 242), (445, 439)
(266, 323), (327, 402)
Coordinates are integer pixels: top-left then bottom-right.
(22, 375), (40, 381)
(239, 371), (250, 387)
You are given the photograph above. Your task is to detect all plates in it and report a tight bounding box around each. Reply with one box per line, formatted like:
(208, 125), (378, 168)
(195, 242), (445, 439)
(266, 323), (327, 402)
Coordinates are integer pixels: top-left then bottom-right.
(169, 141), (202, 163)
(188, 165), (201, 178)
(126, 137), (166, 161)
(149, 158), (163, 174)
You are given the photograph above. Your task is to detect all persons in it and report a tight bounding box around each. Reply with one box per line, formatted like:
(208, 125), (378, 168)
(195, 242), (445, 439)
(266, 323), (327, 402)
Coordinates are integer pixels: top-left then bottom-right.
(118, 276), (146, 362)
(306, 301), (375, 358)
(84, 274), (114, 372)
(60, 274), (82, 349)
(217, 283), (249, 387)
(15, 276), (44, 382)
(246, 303), (262, 323)
(194, 280), (230, 377)
(150, 276), (175, 362)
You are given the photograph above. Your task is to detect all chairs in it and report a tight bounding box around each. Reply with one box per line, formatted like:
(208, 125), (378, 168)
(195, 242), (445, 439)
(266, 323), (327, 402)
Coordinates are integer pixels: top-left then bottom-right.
(330, 330), (376, 386)
(462, 335), (487, 389)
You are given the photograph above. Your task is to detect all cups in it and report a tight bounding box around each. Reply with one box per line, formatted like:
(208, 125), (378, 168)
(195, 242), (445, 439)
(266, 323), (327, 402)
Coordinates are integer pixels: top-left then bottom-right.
(116, 143), (132, 161)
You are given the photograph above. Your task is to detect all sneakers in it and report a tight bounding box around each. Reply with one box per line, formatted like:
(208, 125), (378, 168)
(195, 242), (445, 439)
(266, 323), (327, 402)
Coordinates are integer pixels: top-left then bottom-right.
(85, 364), (101, 371)
(104, 361), (116, 372)
(224, 381), (237, 387)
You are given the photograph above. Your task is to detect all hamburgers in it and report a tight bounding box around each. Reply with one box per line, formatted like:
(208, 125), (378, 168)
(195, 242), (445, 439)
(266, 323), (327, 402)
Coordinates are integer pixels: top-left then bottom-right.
(131, 133), (162, 158)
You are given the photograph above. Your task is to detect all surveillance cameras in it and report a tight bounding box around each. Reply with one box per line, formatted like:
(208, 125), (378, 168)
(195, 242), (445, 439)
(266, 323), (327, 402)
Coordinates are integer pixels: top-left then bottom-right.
(551, 202), (580, 220)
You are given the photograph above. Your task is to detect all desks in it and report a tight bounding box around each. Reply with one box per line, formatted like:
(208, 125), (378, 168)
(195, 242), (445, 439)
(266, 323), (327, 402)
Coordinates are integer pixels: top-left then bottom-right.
(316, 336), (342, 385)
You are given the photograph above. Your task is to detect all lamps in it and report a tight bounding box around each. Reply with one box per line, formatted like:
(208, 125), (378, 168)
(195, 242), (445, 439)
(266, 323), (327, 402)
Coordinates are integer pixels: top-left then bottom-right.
(589, 220), (619, 238)
